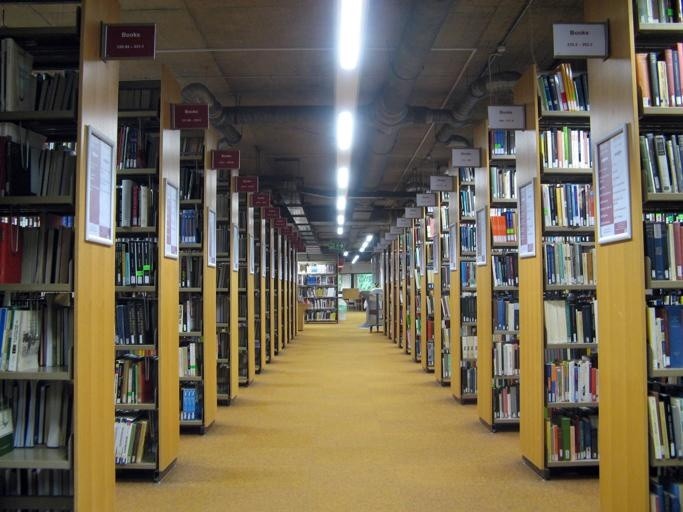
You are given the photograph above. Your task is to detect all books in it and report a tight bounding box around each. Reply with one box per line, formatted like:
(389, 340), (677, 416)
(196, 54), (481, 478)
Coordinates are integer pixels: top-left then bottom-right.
(236, 192), (249, 377)
(117, 84), (157, 228)
(491, 251), (522, 419)
(178, 294), (205, 420)
(633, 0), (683, 512)
(489, 127), (519, 245)
(115, 235), (158, 464)
(1, 377), (76, 497)
(538, 60), (596, 285)
(178, 132), (203, 289)
(542, 291), (599, 462)
(252, 212), (294, 370)
(2, 39), (76, 196)
(1, 211), (77, 374)
(296, 274), (336, 321)
(400, 166), (480, 393)
(216, 169), (230, 395)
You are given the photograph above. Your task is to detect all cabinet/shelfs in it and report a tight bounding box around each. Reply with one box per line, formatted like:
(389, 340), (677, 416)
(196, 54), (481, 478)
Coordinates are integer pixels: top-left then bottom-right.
(0, 0), (338, 512)
(373, 0), (683, 512)
